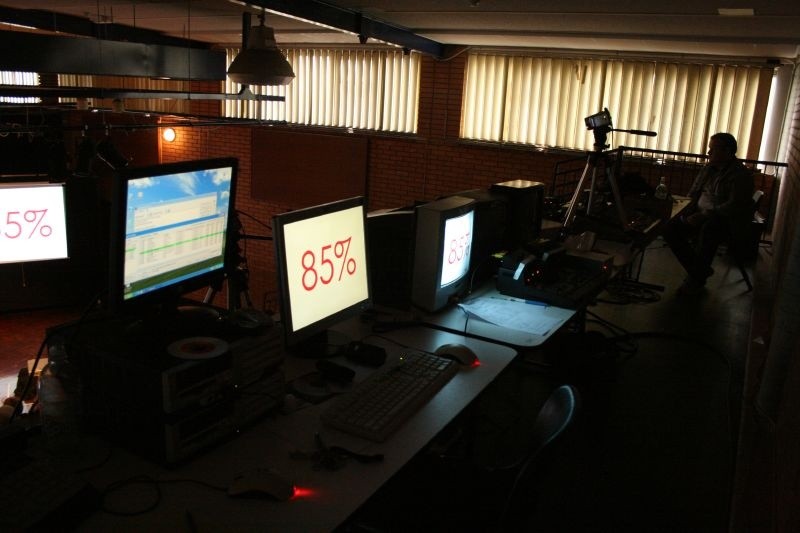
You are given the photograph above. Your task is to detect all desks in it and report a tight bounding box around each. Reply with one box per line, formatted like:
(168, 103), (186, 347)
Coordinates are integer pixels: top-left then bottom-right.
(0, 327), (519, 533)
(413, 191), (693, 380)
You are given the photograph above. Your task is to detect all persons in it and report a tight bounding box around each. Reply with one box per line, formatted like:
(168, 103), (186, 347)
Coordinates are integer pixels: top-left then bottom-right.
(673, 133), (754, 296)
(89, 139), (123, 207)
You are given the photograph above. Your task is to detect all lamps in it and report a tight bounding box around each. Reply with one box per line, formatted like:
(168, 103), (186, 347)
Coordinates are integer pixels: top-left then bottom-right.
(228, 0), (296, 87)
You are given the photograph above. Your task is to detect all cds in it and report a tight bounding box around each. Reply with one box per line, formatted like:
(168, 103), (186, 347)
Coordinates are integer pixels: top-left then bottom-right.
(169, 337), (231, 359)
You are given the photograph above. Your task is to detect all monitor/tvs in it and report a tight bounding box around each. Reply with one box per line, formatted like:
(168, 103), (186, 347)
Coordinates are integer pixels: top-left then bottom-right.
(365, 189), (508, 314)
(270, 196), (371, 360)
(109, 156), (238, 310)
(1, 174), (69, 266)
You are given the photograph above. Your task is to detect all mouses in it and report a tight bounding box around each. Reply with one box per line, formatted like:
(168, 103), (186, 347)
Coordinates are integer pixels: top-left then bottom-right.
(230, 472), (291, 502)
(432, 344), (477, 365)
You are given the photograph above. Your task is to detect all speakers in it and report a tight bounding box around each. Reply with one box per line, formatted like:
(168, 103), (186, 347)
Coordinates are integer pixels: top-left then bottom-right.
(491, 180), (544, 251)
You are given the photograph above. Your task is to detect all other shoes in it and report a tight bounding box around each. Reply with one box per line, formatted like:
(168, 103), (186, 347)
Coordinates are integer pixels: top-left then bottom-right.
(684, 266), (715, 282)
(677, 279), (704, 296)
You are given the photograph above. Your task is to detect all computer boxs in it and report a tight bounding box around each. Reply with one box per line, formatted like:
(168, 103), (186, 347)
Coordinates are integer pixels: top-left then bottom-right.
(0, 239), (82, 311)
(46, 298), (271, 444)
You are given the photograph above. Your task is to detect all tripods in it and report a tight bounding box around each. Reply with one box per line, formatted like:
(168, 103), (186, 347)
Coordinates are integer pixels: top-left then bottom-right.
(563, 155), (627, 231)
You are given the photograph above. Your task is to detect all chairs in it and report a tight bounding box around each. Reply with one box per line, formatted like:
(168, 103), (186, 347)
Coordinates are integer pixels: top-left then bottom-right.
(398, 384), (582, 532)
(721, 191), (766, 291)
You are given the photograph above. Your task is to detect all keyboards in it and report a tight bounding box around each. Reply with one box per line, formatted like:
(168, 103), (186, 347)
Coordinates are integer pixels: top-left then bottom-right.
(319, 352), (460, 446)
